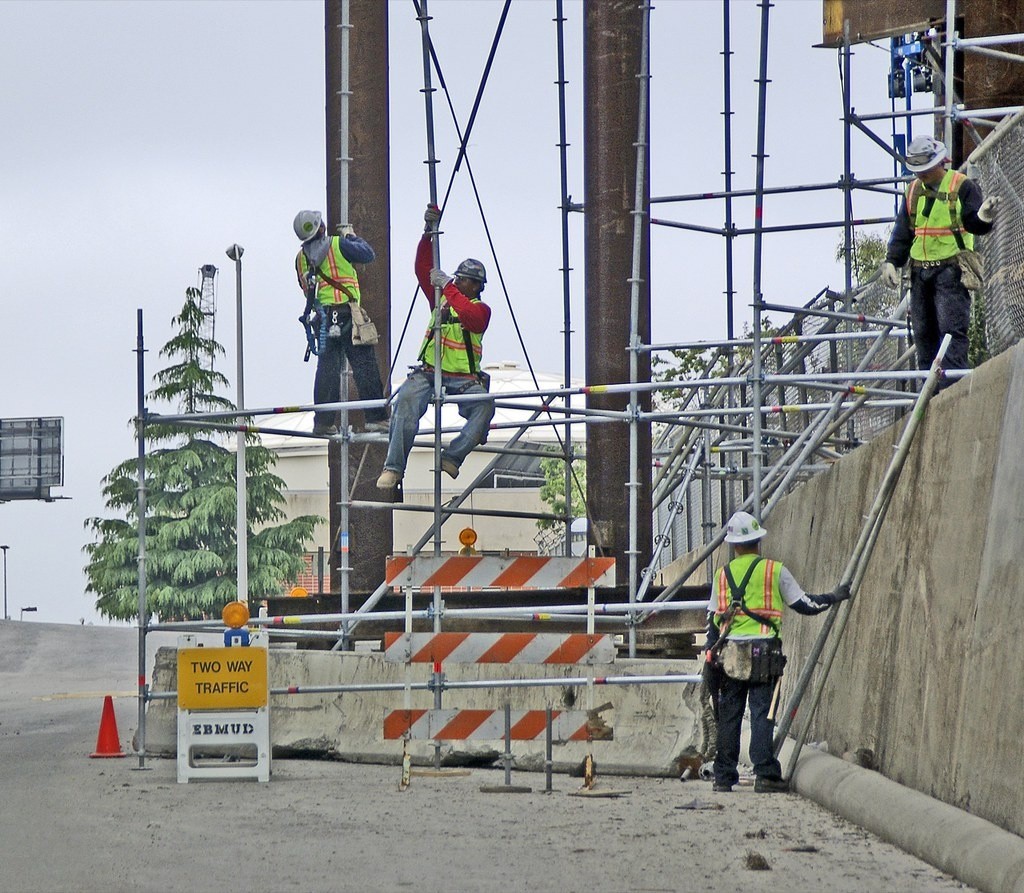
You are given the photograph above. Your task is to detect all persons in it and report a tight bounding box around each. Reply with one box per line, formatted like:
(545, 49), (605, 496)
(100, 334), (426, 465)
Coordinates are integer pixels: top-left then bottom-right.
(293, 210), (391, 434)
(702, 512), (854, 791)
(883, 136), (1002, 399)
(376, 202), (494, 488)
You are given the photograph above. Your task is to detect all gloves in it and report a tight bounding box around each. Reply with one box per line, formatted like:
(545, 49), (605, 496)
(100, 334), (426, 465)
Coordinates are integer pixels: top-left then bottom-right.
(883, 262), (899, 290)
(423, 202), (440, 231)
(977, 195), (1004, 223)
(703, 647), (719, 663)
(336, 224), (356, 239)
(832, 580), (853, 602)
(429, 268), (455, 289)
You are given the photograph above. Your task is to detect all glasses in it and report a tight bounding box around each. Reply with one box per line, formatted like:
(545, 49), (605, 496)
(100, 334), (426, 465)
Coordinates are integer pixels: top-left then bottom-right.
(906, 153), (931, 166)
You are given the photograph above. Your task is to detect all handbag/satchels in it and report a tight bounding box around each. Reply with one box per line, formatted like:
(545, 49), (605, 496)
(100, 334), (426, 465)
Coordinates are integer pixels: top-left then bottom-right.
(348, 301), (379, 345)
(958, 249), (986, 291)
(722, 639), (753, 681)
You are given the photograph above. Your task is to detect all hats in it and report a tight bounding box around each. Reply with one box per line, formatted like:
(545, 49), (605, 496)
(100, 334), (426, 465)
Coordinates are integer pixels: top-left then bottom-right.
(905, 135), (947, 173)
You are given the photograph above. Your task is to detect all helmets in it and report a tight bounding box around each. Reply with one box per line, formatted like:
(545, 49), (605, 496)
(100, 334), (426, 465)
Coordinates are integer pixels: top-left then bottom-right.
(293, 210), (322, 247)
(723, 512), (767, 543)
(453, 259), (487, 284)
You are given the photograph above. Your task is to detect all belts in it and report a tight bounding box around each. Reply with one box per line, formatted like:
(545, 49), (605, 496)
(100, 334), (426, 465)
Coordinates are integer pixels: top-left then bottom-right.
(912, 255), (957, 269)
(425, 365), (477, 381)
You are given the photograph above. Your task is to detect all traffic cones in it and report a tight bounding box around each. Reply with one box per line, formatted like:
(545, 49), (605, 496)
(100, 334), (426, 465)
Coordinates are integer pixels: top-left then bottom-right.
(89, 695), (127, 758)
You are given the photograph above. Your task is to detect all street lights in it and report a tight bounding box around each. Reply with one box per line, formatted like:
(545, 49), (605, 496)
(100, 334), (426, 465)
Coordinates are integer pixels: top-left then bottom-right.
(225, 242), (243, 604)
(0, 545), (9, 619)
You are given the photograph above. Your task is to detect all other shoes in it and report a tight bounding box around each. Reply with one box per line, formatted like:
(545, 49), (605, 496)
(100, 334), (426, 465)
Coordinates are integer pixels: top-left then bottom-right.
(754, 775), (789, 793)
(313, 422), (339, 436)
(364, 416), (390, 432)
(713, 782), (732, 792)
(439, 452), (459, 479)
(376, 468), (404, 488)
